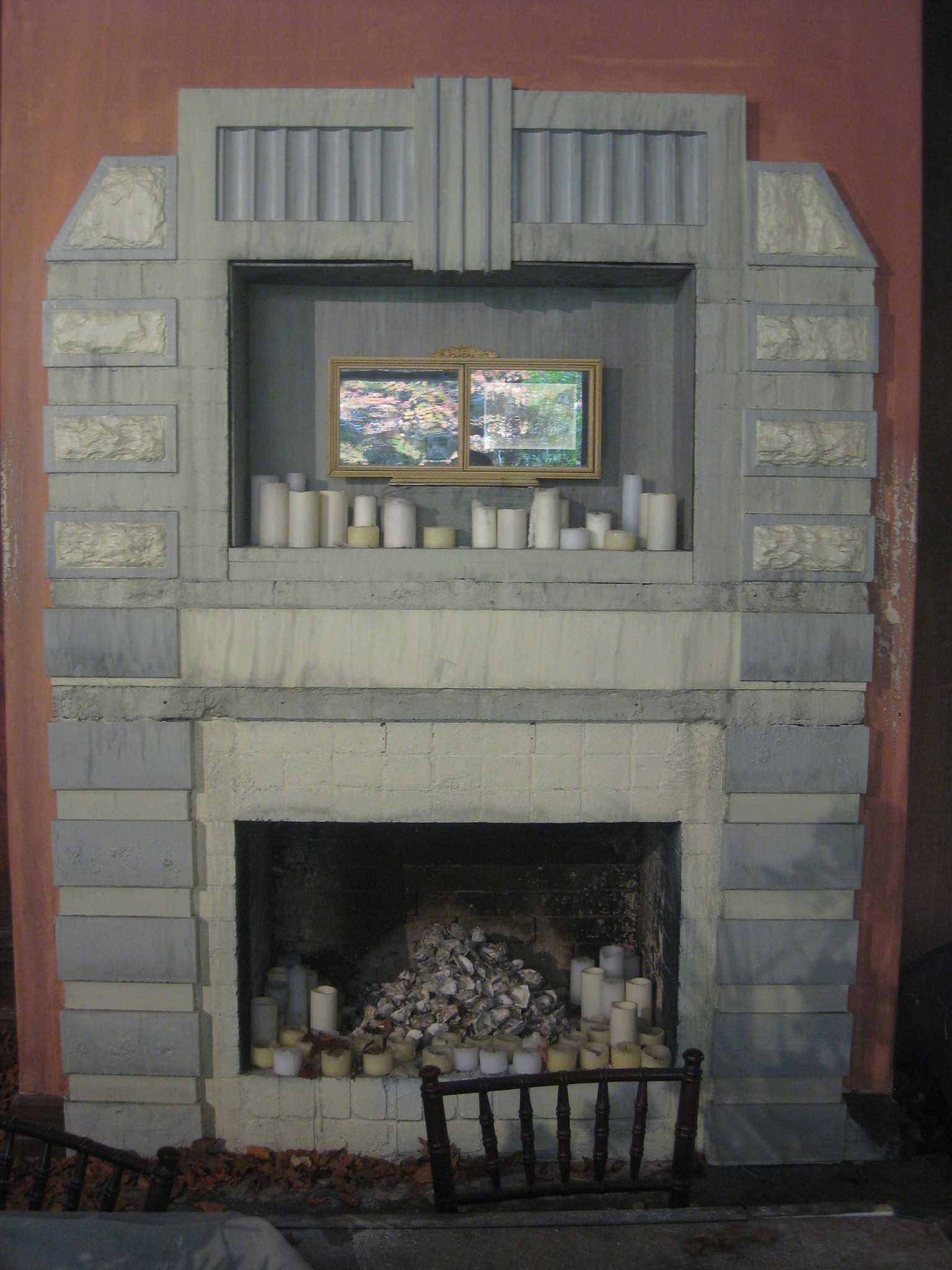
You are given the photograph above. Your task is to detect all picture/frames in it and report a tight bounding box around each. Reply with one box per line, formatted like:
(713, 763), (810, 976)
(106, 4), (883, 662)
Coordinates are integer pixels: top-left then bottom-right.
(334, 362), (595, 472)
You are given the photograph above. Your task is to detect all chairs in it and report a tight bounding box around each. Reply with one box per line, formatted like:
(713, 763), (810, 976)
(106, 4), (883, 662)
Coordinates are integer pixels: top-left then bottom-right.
(0, 1116), (180, 1212)
(419, 1049), (705, 1215)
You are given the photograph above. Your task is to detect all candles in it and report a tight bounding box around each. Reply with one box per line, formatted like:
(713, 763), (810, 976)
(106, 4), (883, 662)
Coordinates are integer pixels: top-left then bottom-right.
(248, 945), (673, 1076)
(250, 472), (676, 551)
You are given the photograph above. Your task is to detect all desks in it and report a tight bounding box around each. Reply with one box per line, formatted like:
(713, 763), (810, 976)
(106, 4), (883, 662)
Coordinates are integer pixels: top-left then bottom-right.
(261, 1204), (951, 1268)
